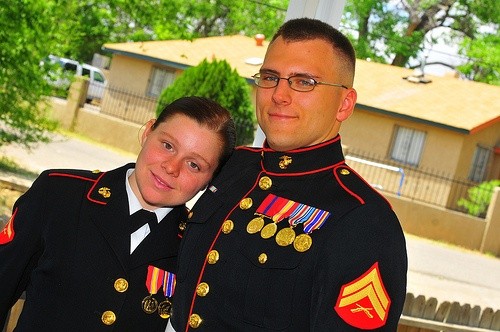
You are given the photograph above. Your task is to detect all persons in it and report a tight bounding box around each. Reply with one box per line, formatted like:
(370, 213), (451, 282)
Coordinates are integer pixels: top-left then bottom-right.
(166, 17), (408, 332)
(0, 96), (235, 332)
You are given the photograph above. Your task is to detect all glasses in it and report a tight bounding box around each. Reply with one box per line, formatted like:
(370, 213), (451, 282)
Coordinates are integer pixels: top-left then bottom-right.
(251, 71), (349, 93)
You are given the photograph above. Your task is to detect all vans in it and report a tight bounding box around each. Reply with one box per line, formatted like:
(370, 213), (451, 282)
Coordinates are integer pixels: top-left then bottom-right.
(45, 58), (105, 103)
